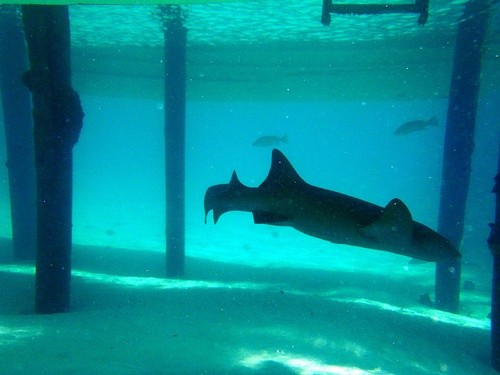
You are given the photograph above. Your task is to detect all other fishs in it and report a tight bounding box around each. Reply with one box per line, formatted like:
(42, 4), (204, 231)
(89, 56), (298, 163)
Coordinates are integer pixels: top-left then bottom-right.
(394, 116), (438, 137)
(204, 149), (461, 267)
(252, 133), (290, 147)
